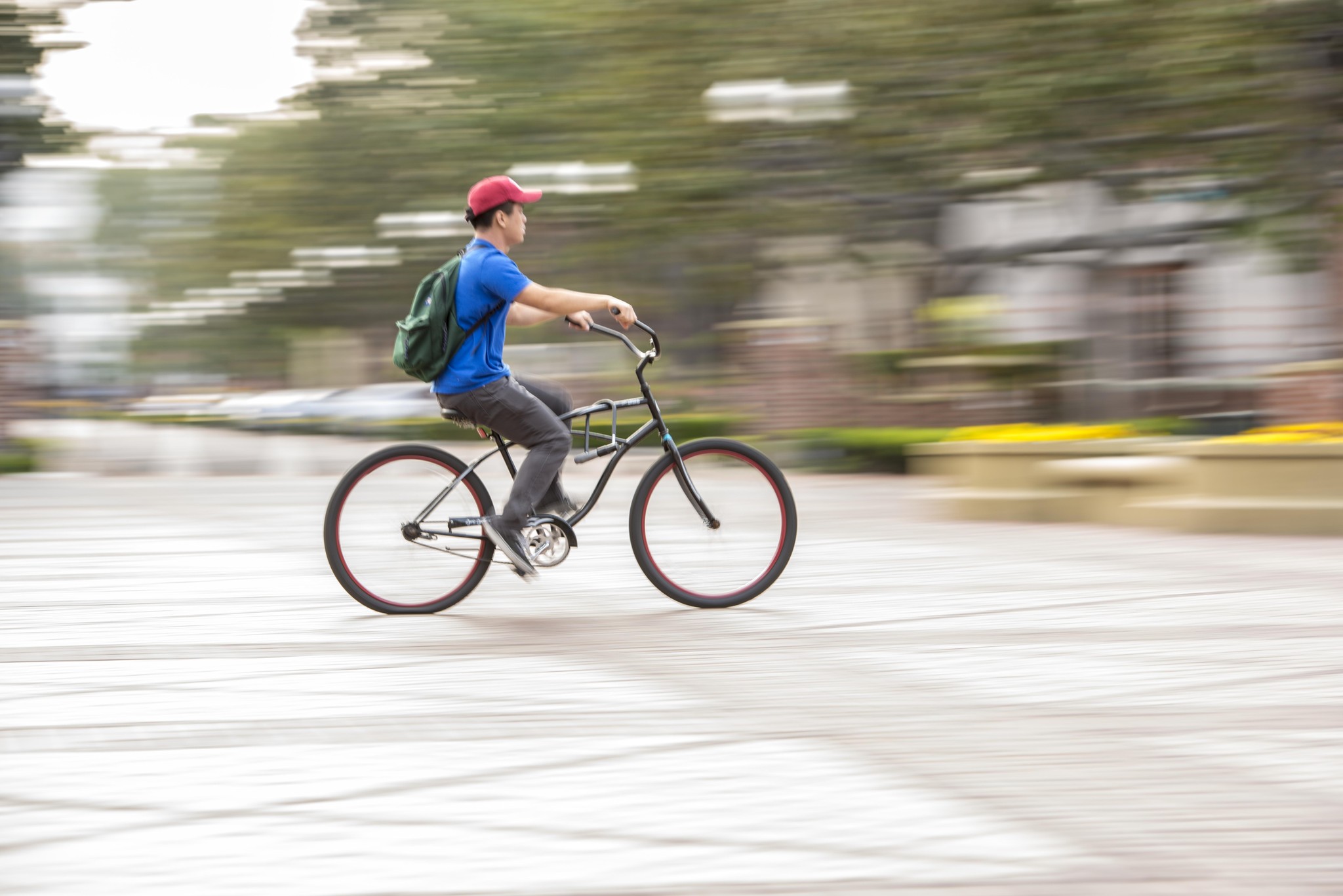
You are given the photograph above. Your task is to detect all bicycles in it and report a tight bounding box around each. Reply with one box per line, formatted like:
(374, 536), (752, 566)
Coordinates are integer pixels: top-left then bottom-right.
(320, 305), (799, 615)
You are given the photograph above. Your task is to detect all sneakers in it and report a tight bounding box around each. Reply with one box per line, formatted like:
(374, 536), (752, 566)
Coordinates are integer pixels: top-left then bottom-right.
(537, 498), (584, 520)
(482, 515), (536, 577)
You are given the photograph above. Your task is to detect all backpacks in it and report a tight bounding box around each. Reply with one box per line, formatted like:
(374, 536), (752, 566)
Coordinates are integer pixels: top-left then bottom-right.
(393, 248), (506, 382)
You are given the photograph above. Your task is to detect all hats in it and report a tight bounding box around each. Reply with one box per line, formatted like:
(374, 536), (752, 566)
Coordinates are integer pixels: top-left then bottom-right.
(463, 176), (542, 220)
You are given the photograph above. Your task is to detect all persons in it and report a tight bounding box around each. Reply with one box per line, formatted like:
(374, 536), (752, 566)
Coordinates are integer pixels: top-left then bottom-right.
(432, 176), (641, 581)
